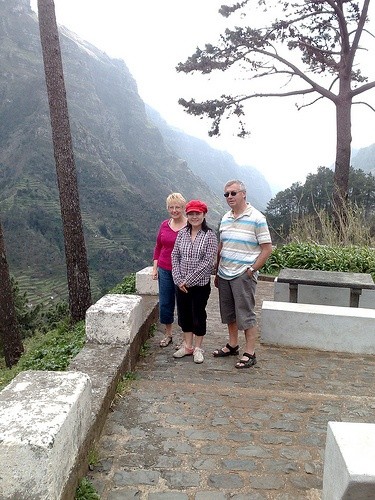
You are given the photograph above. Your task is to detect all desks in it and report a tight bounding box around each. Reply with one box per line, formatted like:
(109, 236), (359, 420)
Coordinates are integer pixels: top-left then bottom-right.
(277, 267), (375, 308)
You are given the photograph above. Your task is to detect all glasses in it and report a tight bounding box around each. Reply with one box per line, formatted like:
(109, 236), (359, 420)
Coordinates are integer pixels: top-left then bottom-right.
(223, 190), (243, 197)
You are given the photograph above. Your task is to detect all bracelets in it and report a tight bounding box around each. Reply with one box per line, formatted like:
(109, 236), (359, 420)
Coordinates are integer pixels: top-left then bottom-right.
(250, 267), (256, 274)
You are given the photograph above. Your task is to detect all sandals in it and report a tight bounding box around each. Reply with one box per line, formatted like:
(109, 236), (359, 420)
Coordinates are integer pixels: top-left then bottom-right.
(212, 343), (239, 357)
(235, 353), (257, 369)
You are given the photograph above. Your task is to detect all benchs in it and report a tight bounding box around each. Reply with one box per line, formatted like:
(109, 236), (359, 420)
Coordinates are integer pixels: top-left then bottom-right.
(273, 276), (375, 311)
(260, 299), (375, 355)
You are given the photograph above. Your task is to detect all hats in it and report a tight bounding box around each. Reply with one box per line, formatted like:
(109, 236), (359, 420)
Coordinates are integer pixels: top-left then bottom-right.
(184, 200), (207, 215)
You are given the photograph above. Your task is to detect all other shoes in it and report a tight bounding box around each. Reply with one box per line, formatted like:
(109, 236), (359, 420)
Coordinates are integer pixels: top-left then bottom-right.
(173, 342), (194, 357)
(159, 334), (173, 346)
(193, 347), (205, 363)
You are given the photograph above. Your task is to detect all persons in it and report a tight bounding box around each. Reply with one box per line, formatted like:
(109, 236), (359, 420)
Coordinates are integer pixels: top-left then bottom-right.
(152, 192), (187, 351)
(172, 200), (217, 363)
(213, 180), (272, 369)
